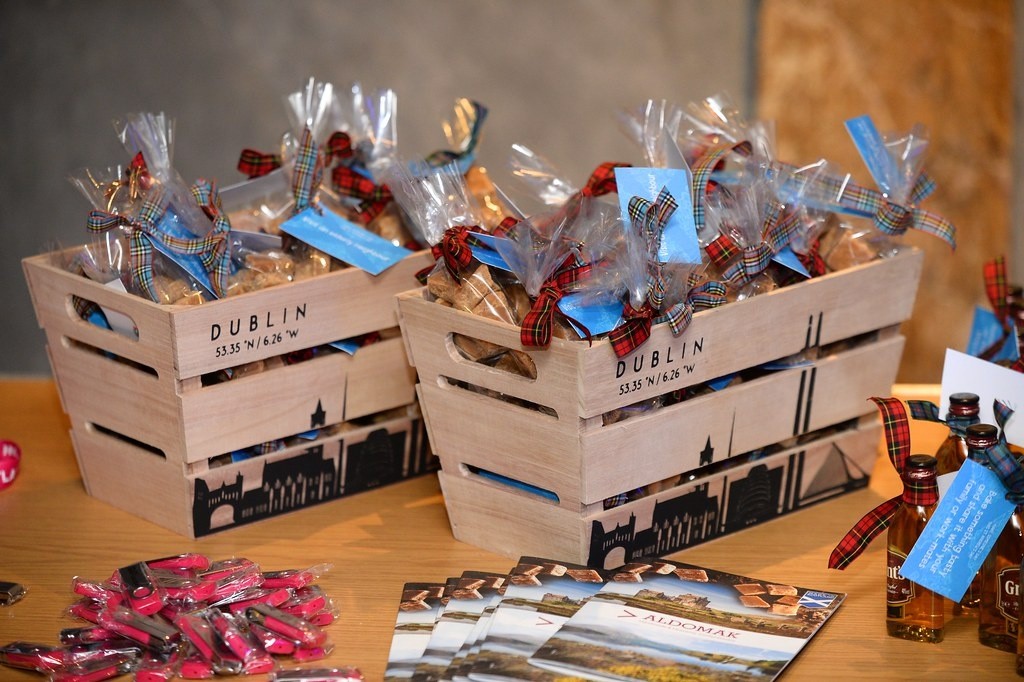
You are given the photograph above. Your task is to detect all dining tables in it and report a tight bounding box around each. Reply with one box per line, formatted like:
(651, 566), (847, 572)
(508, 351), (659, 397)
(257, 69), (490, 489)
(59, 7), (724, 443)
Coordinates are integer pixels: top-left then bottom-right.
(0, 378), (1024, 682)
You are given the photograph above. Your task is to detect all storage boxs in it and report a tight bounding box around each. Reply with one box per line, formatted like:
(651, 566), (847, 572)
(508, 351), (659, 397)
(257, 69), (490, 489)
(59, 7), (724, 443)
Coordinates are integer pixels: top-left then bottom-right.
(394, 244), (923, 572)
(21, 204), (442, 539)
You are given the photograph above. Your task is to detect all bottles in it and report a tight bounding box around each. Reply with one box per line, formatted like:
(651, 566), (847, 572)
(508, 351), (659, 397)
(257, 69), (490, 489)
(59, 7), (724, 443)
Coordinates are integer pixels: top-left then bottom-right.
(886, 393), (1024, 678)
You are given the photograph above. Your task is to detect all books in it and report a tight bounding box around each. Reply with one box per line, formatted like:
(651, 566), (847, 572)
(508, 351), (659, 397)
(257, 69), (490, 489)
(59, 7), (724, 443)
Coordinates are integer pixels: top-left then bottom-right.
(382, 554), (850, 682)
